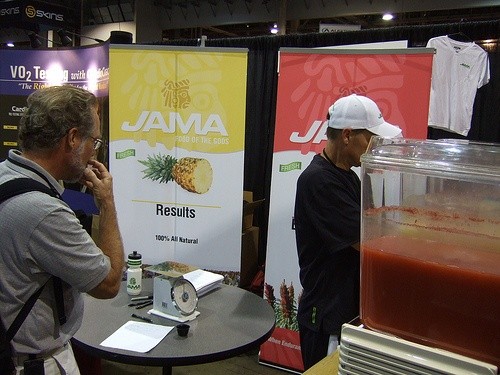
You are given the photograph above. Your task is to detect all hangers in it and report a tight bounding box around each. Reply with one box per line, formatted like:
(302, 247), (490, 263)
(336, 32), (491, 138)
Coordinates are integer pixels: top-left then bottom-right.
(447, 17), (473, 42)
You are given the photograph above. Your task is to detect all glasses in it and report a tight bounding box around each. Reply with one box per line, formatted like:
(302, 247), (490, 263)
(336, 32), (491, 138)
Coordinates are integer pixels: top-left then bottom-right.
(77, 130), (103, 150)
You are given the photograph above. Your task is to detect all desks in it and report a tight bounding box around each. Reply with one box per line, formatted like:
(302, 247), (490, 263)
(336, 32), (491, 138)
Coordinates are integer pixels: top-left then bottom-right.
(69, 278), (275, 375)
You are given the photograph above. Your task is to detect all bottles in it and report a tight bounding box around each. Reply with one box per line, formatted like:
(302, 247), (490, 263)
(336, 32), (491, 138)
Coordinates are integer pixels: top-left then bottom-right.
(125, 251), (143, 296)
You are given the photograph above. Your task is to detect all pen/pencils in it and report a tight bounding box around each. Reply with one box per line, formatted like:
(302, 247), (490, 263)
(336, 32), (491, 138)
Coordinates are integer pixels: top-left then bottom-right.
(127, 300), (151, 306)
(131, 296), (153, 300)
(137, 301), (153, 308)
(130, 313), (153, 322)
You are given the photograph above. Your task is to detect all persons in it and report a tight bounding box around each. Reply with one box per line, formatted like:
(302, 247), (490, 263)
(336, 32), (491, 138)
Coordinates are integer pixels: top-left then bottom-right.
(0, 84), (125, 375)
(294, 94), (402, 370)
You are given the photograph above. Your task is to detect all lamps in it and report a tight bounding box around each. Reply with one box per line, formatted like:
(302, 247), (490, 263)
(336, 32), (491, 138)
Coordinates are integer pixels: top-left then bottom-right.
(27, 30), (62, 48)
(57, 28), (105, 45)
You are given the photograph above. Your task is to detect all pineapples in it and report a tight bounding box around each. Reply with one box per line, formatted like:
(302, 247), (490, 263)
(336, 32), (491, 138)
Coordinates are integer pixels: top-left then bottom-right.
(136, 152), (212, 194)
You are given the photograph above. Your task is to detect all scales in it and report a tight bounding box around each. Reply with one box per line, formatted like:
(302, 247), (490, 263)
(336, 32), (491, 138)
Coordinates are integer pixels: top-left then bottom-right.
(144, 261), (200, 323)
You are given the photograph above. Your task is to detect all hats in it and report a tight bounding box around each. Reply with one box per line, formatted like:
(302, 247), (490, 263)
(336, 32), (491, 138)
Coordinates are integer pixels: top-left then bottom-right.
(326, 93), (403, 140)
